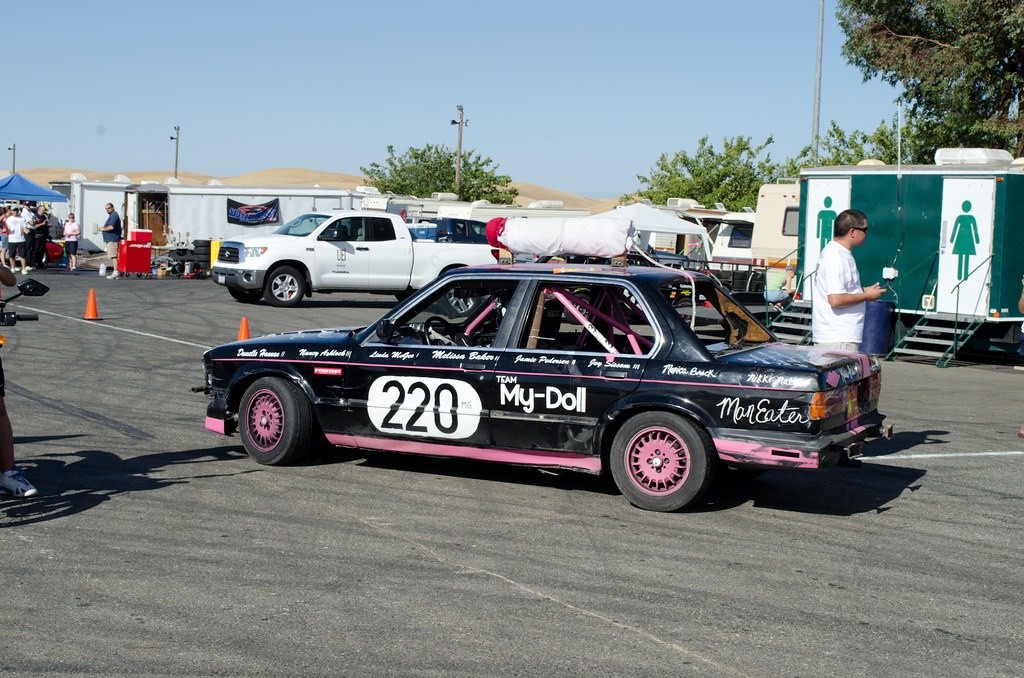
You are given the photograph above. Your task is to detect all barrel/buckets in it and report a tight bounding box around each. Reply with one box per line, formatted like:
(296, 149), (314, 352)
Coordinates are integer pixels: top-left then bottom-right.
(860, 301), (895, 353)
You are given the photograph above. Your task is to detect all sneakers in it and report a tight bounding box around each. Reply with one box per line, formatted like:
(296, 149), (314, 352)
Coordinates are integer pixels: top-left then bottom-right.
(0, 466), (38, 498)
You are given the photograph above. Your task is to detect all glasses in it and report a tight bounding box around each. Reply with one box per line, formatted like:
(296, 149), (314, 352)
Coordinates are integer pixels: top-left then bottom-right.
(852, 226), (868, 232)
(105, 207), (110, 209)
(69, 217), (74, 218)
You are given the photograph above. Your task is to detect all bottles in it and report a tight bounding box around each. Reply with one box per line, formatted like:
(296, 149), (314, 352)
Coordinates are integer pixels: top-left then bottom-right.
(99, 263), (106, 276)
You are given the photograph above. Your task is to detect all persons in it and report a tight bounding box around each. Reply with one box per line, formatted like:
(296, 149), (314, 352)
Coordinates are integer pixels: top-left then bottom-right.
(762, 264), (801, 314)
(0, 201), (81, 276)
(0, 286), (39, 502)
(97, 202), (123, 281)
(809, 208), (886, 477)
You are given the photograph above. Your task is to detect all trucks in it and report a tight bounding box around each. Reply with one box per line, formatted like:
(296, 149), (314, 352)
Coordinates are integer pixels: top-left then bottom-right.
(616, 178), (800, 292)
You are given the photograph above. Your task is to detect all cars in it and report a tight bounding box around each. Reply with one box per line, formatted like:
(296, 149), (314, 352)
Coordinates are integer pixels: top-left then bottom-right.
(534, 243), (708, 325)
(190, 265), (894, 514)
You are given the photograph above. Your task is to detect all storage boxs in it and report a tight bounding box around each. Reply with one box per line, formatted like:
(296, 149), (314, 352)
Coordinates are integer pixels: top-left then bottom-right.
(131, 229), (152, 242)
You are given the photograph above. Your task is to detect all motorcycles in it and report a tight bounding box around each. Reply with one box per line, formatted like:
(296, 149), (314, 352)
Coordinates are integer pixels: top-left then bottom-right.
(0, 278), (50, 348)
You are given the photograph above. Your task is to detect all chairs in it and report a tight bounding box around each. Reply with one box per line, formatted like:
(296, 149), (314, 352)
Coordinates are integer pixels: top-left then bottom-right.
(338, 225), (348, 240)
(348, 226), (363, 241)
(539, 300), (564, 342)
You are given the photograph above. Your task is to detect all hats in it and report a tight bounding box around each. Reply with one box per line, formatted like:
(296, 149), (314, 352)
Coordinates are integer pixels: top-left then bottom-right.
(11, 205), (18, 210)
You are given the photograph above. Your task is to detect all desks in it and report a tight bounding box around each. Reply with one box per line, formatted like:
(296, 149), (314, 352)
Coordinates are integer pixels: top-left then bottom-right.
(152, 246), (195, 256)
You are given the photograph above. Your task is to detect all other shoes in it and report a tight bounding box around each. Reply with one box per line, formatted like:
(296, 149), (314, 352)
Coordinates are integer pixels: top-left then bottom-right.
(15, 267), (20, 271)
(70, 267), (76, 271)
(22, 270), (28, 275)
(3, 265), (9, 268)
(106, 273), (119, 280)
(26, 266), (32, 271)
(11, 268), (16, 273)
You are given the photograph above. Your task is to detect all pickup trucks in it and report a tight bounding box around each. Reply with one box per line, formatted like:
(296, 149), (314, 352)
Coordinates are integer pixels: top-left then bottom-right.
(211, 210), (516, 307)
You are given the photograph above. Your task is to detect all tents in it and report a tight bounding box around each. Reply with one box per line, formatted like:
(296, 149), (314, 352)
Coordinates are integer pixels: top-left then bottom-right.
(0, 170), (70, 219)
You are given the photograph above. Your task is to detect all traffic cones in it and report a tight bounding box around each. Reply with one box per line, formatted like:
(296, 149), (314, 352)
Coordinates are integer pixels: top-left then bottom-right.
(81, 288), (104, 321)
(237, 317), (250, 342)
